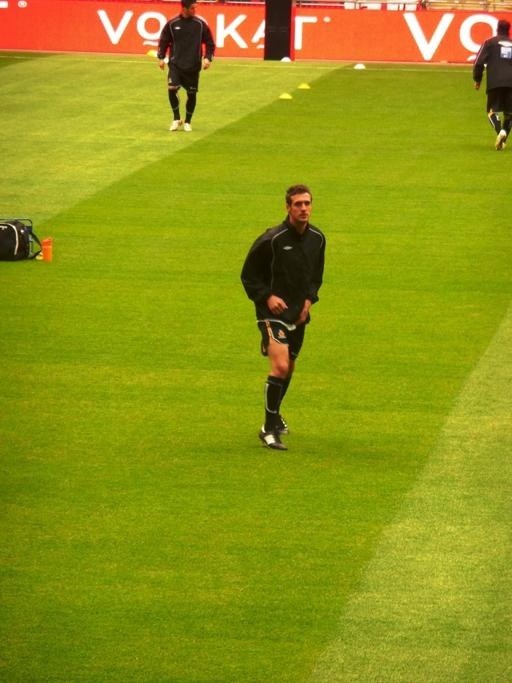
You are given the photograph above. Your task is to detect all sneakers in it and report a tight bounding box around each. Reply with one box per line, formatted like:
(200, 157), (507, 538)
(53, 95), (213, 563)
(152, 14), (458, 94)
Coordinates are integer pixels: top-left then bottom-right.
(494, 129), (507, 149)
(276, 416), (290, 435)
(258, 424), (288, 450)
(183, 121), (192, 132)
(169, 118), (182, 130)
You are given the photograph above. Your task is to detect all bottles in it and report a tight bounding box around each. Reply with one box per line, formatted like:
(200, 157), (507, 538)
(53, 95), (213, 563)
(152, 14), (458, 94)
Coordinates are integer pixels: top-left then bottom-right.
(41, 237), (52, 262)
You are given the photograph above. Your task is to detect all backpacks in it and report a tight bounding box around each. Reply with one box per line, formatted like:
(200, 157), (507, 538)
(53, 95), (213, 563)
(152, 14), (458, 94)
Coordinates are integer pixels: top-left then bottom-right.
(0, 217), (44, 262)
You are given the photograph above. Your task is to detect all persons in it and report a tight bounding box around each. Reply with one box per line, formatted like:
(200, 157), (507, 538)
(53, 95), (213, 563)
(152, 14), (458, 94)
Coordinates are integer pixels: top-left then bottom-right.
(156, 0), (215, 130)
(472, 18), (512, 151)
(240, 183), (325, 451)
